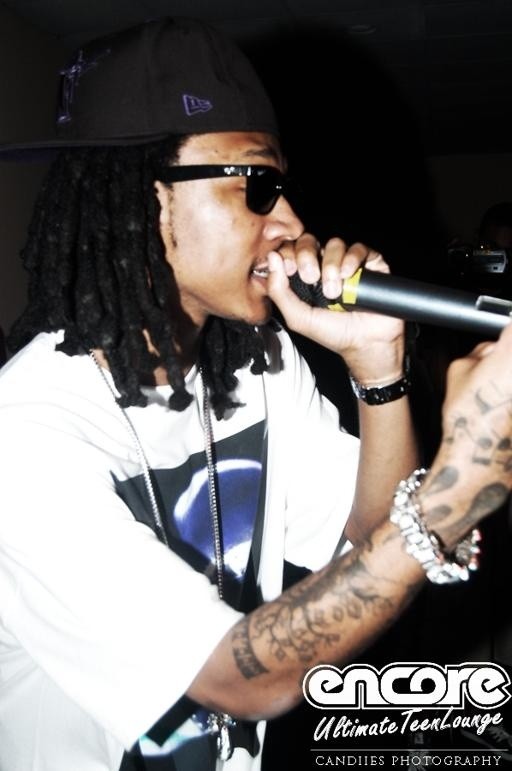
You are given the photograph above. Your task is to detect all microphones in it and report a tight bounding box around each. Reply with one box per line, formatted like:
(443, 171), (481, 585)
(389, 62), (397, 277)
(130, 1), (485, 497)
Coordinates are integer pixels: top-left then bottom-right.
(287, 253), (512, 346)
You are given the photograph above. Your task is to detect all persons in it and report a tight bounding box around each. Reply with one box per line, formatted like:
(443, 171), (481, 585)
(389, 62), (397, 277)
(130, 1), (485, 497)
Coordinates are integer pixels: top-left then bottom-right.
(0, 14), (512, 771)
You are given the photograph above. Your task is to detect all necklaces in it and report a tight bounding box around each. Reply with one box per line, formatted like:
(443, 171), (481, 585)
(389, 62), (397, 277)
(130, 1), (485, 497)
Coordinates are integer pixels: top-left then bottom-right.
(86, 349), (236, 761)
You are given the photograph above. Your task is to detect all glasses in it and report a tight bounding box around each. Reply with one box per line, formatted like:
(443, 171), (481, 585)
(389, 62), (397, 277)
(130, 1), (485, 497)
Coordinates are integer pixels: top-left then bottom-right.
(160, 164), (290, 216)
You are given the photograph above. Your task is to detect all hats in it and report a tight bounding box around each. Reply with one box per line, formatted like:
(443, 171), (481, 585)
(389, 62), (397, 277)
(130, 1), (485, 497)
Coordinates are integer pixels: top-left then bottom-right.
(0, 15), (280, 163)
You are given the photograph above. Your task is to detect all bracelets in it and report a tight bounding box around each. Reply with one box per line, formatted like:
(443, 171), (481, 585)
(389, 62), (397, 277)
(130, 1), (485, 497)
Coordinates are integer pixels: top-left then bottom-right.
(389, 469), (483, 585)
(349, 376), (412, 406)
